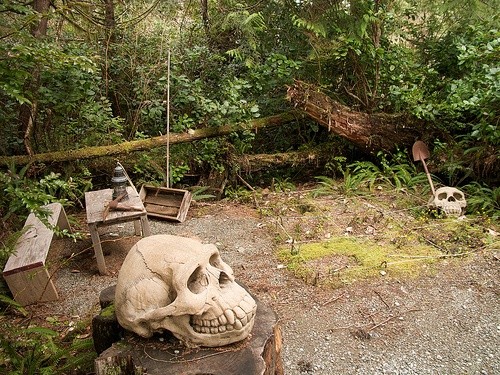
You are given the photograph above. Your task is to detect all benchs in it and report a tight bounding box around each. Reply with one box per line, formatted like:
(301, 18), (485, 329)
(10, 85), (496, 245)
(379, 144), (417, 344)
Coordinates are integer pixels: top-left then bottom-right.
(3, 202), (72, 308)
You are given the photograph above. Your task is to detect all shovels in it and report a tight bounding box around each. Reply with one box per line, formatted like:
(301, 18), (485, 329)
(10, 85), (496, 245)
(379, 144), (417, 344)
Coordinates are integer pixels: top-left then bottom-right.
(412, 141), (436, 195)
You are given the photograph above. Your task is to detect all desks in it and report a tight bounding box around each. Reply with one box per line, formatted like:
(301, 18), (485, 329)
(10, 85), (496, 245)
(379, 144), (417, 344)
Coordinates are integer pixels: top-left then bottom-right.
(85, 186), (149, 276)
